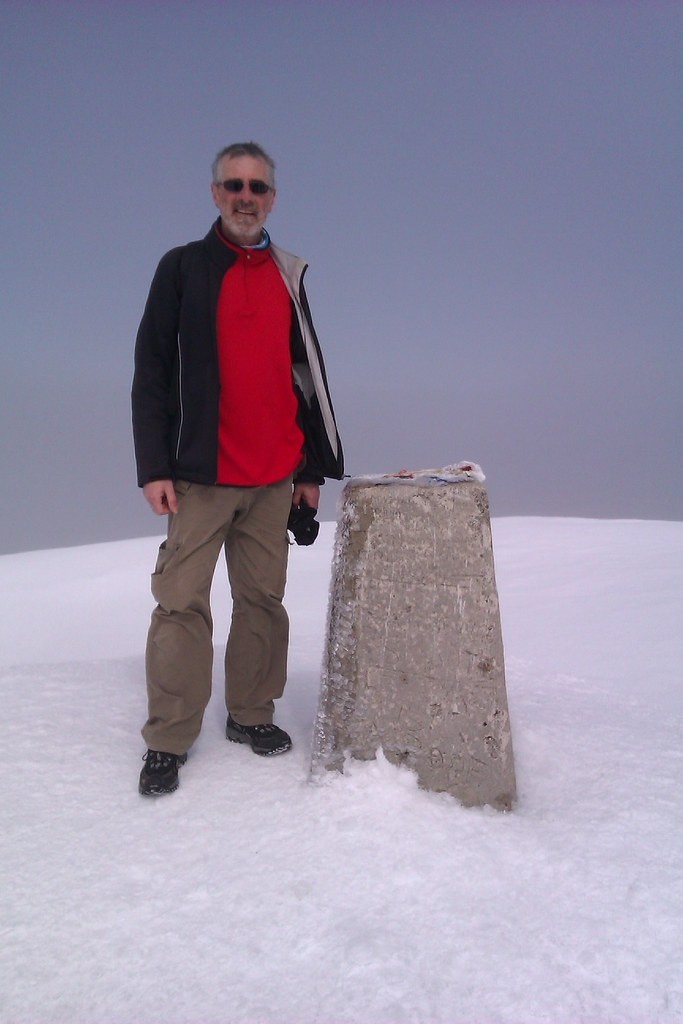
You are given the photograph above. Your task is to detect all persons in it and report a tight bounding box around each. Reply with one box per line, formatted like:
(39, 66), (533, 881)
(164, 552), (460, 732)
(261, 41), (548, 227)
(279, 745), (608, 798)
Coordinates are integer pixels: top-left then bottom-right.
(131, 140), (347, 798)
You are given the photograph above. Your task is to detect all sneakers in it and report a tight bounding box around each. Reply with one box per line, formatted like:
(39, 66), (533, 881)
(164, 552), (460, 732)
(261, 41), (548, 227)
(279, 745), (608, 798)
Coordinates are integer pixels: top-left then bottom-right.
(225, 711), (291, 756)
(138, 749), (188, 796)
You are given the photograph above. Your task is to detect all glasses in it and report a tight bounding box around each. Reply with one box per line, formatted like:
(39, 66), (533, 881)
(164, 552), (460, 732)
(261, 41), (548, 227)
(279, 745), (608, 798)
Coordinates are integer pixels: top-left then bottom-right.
(215, 178), (274, 194)
